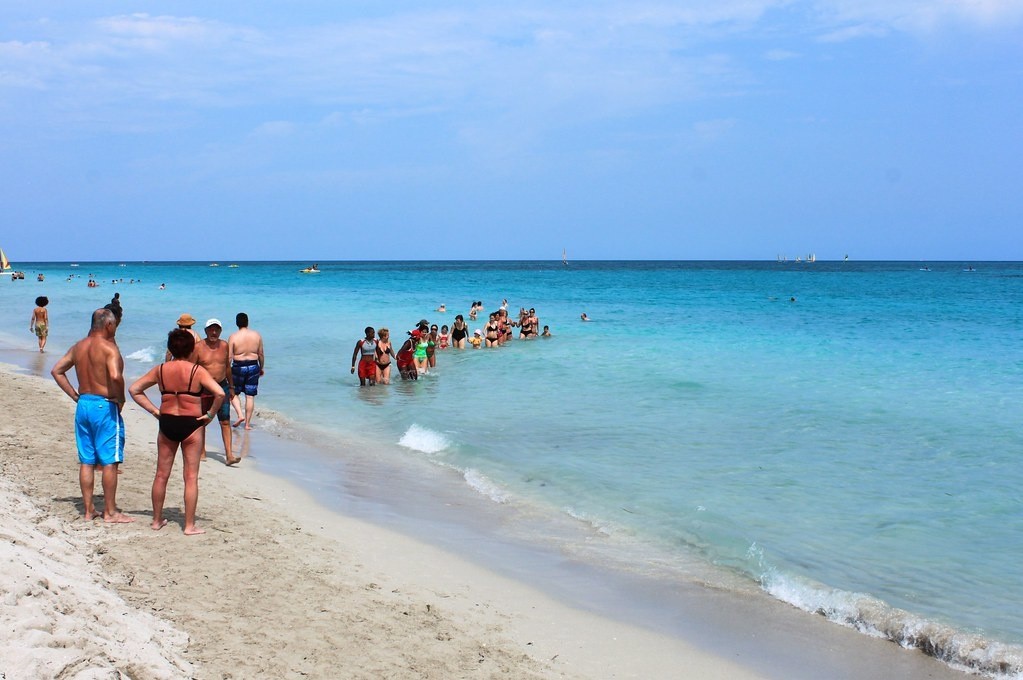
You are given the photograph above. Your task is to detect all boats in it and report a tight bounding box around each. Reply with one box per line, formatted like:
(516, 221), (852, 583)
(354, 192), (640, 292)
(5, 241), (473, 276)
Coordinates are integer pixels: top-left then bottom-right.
(209, 263), (219, 267)
(298, 269), (321, 274)
(228, 264), (240, 268)
(119, 264), (127, 267)
(71, 264), (79, 267)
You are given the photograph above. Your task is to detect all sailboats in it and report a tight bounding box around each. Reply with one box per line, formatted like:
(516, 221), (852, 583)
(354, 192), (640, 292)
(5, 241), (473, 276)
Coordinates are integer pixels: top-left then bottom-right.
(0, 248), (11, 270)
(777, 252), (816, 264)
(561, 247), (569, 265)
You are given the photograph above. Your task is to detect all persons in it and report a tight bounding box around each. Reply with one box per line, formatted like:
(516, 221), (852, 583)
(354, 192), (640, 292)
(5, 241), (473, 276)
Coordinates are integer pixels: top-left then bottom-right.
(581, 313), (588, 320)
(396, 307), (551, 380)
(228, 313), (264, 429)
(37, 273), (44, 281)
(51, 293), (138, 522)
(165, 314), (200, 361)
(129, 329), (225, 534)
(503, 299), (508, 307)
(11, 270), (24, 280)
(160, 283), (165, 289)
(439, 304), (445, 310)
(88, 273), (140, 287)
(375, 329), (396, 384)
(469, 301), (483, 320)
(30, 296), (49, 352)
(351, 327), (380, 386)
(190, 319), (241, 466)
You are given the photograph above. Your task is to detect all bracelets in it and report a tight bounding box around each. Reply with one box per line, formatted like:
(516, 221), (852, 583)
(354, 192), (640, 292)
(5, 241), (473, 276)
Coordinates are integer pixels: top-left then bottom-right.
(207, 412), (214, 419)
(352, 367), (355, 368)
(230, 385), (235, 388)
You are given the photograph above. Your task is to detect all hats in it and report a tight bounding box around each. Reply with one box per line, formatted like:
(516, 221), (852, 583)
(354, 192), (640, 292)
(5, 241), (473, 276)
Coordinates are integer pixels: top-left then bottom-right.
(205, 319), (223, 333)
(420, 320), (430, 325)
(412, 330), (424, 340)
(176, 314), (196, 326)
(474, 329), (481, 336)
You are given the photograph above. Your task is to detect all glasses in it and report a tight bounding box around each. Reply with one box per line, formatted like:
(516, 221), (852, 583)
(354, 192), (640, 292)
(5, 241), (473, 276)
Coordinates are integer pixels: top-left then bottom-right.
(431, 328), (438, 332)
(523, 313), (528, 316)
(424, 323), (428, 325)
(530, 311), (534, 313)
